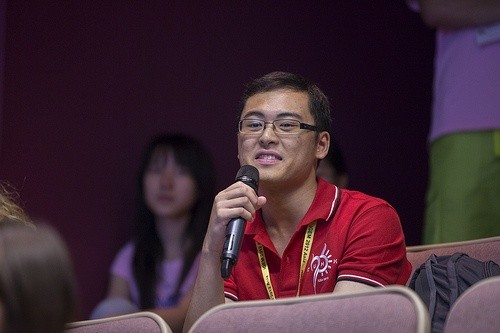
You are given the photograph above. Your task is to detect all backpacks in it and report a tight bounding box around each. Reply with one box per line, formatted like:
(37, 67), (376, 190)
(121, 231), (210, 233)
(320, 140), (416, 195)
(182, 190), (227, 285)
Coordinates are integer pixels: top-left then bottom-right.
(408, 252), (500, 333)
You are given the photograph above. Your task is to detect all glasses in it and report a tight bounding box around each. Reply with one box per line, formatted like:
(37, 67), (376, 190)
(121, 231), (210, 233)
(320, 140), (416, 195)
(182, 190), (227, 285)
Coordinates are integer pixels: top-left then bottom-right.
(238, 118), (320, 135)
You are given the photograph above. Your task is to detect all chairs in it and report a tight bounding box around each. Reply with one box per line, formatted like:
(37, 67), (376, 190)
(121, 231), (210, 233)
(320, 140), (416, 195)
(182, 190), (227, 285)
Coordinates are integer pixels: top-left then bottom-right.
(406, 237), (499, 287)
(66, 276), (500, 333)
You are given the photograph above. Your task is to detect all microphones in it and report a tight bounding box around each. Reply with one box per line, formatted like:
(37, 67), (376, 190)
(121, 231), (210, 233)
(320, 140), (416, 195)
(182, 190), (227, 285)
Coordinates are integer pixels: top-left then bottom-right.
(221, 165), (259, 279)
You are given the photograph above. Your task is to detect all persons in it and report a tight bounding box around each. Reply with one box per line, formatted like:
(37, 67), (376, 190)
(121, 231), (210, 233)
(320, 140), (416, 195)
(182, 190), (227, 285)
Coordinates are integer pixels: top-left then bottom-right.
(314, 137), (349, 191)
(90, 131), (212, 333)
(409, 1), (500, 246)
(181, 70), (413, 332)
(0, 219), (79, 333)
